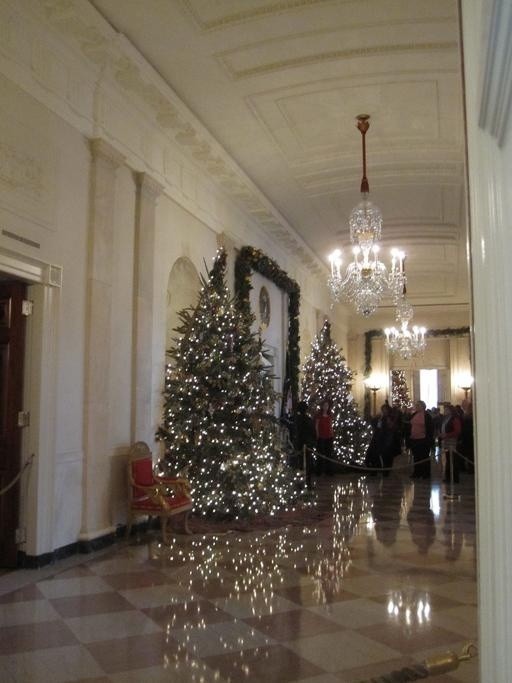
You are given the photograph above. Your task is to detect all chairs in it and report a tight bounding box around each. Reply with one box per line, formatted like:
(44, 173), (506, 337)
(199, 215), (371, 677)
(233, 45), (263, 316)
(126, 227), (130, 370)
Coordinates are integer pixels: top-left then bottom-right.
(120, 441), (198, 546)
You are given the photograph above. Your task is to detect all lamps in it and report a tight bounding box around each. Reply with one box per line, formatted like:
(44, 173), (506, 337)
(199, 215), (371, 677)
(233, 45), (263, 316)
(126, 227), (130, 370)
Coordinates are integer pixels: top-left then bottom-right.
(328, 113), (431, 361)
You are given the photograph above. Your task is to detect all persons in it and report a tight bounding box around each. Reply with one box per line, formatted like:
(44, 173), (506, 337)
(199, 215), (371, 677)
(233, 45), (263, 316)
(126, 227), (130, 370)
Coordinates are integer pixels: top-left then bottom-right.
(365, 400), (474, 484)
(295, 401), (316, 474)
(366, 476), (475, 561)
(314, 399), (335, 477)
